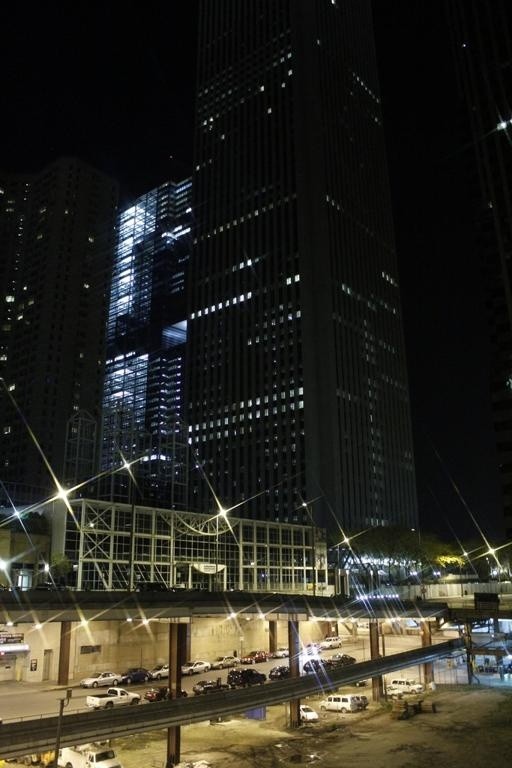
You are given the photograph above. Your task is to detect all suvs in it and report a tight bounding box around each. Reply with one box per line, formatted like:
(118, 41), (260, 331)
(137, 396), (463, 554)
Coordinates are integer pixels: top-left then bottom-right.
(227, 668), (266, 688)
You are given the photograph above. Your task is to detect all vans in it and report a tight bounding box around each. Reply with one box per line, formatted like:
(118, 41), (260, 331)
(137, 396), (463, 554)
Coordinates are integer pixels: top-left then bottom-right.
(299, 705), (319, 722)
(390, 679), (424, 694)
(320, 694), (368, 713)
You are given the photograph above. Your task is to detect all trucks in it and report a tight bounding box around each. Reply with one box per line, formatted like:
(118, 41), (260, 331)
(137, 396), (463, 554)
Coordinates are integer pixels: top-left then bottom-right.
(58, 742), (122, 768)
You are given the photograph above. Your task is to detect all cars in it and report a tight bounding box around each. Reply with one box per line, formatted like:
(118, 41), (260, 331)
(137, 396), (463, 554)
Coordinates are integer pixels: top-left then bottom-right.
(276, 648), (289, 658)
(144, 686), (187, 702)
(211, 655), (240, 669)
(241, 651), (267, 665)
(181, 660), (212, 676)
(269, 665), (291, 680)
(80, 664), (170, 689)
(304, 654), (356, 672)
(301, 637), (342, 655)
(193, 680), (229, 695)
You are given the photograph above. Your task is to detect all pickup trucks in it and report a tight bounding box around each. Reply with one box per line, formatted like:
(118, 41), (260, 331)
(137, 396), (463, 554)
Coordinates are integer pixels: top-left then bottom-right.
(87, 687), (141, 710)
